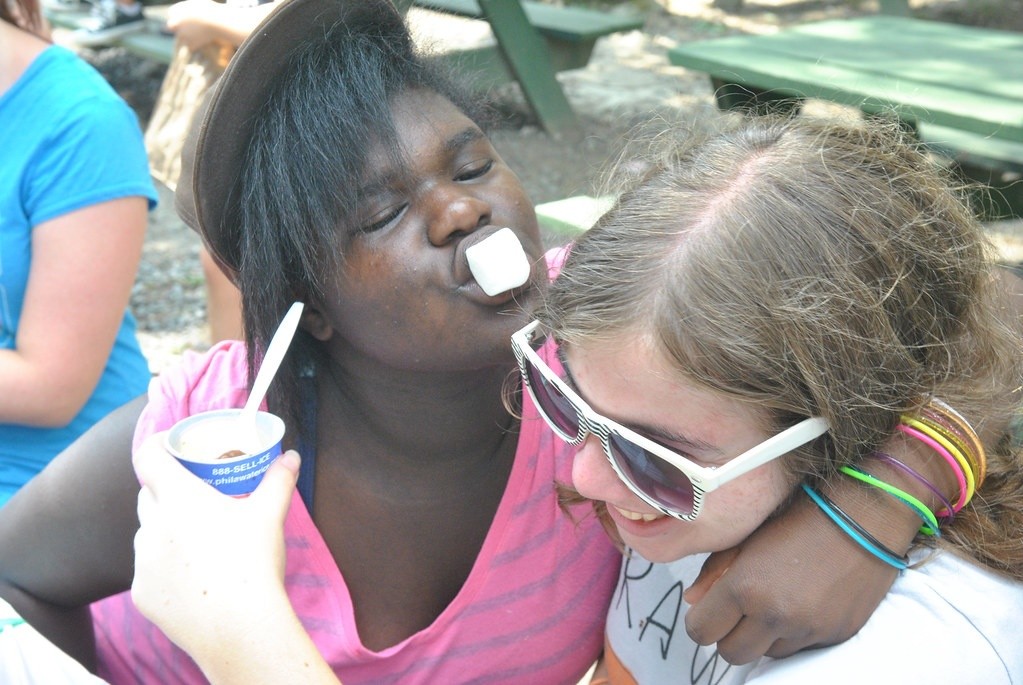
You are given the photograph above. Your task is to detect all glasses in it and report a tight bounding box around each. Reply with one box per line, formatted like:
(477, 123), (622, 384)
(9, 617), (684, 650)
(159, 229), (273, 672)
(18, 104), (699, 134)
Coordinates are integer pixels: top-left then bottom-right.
(510, 317), (832, 520)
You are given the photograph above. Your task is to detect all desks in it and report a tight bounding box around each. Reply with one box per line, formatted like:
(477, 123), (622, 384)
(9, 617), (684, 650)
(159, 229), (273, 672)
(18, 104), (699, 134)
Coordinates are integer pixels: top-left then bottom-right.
(669, 14), (1023, 227)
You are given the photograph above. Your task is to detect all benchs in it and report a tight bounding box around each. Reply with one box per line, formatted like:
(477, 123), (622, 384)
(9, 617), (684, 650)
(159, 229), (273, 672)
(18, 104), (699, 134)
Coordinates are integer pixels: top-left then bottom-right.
(390, 1), (648, 146)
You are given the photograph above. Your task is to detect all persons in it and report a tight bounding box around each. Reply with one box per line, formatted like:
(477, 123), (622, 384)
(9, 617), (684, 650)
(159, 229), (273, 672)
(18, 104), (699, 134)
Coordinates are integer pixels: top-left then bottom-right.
(1, 1), (1022, 685)
(130, 109), (1023, 685)
(145, 1), (282, 345)
(1, 2), (156, 507)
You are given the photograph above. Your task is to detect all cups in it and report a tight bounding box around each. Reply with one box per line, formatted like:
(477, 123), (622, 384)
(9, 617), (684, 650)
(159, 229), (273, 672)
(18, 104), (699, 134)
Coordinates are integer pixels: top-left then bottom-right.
(165, 408), (286, 498)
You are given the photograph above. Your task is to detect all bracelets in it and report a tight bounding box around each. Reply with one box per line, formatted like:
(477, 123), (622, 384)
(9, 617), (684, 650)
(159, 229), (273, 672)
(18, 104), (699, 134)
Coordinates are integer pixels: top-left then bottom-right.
(801, 393), (986, 570)
